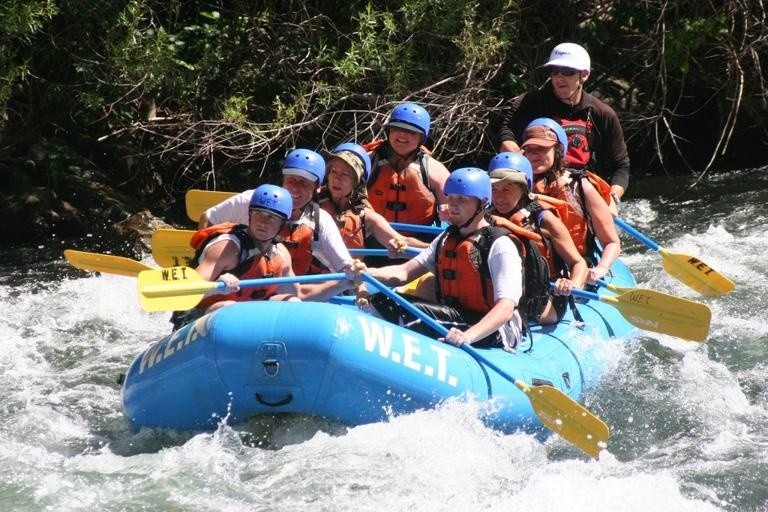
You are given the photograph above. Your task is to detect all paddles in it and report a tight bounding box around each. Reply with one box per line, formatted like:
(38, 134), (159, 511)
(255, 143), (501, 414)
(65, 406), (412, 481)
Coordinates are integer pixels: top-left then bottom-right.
(543, 275), (711, 343)
(184, 189), (446, 234)
(613, 216), (734, 296)
(152, 230), (407, 268)
(138, 265), (357, 312)
(348, 260), (609, 462)
(65, 250), (154, 277)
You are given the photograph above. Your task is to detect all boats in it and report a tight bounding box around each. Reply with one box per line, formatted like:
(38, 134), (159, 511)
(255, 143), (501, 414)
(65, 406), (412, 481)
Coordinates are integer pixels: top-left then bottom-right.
(118, 237), (641, 450)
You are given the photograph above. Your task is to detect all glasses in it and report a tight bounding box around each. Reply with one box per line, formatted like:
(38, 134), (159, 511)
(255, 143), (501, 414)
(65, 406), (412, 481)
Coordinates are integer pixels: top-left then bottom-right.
(550, 67), (575, 76)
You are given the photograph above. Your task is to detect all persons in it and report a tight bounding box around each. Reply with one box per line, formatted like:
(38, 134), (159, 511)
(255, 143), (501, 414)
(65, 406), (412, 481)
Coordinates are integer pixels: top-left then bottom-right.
(346, 168), (522, 347)
(361, 103), (451, 239)
(170, 149), (368, 332)
(386, 152), (590, 325)
(494, 41), (631, 219)
(520, 118), (621, 286)
(314, 143), (431, 273)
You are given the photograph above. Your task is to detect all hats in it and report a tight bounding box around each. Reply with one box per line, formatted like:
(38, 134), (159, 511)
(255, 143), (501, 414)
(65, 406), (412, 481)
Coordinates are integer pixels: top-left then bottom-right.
(541, 42), (591, 72)
(283, 168), (318, 183)
(382, 121), (423, 135)
(320, 148), (365, 182)
(521, 125), (557, 149)
(489, 168), (529, 187)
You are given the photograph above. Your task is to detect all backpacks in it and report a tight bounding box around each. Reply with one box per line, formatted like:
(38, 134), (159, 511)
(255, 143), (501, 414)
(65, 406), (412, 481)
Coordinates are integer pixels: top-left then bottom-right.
(476, 226), (551, 352)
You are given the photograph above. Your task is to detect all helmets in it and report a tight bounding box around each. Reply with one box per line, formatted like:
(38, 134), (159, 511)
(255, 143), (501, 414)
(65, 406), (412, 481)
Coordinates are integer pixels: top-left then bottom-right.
(443, 166), (492, 206)
(334, 142), (372, 182)
(248, 183), (294, 221)
(389, 104), (432, 138)
(487, 151), (535, 191)
(527, 117), (569, 157)
(282, 148), (326, 183)
